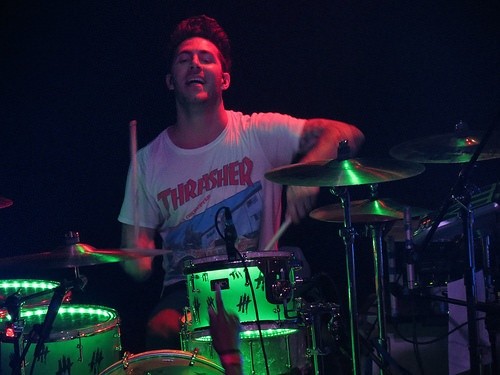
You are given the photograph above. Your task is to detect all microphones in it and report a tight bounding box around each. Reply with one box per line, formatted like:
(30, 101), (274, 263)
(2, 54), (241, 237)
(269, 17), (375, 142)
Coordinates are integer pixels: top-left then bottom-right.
(386, 235), (398, 318)
(224, 207), (239, 242)
(33, 287), (68, 357)
(403, 207), (415, 291)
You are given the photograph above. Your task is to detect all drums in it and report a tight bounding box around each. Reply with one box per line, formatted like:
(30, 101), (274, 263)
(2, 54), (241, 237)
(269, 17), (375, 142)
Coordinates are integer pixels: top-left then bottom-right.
(0, 304), (123, 375)
(184, 250), (304, 341)
(98, 350), (226, 375)
(0, 279), (62, 306)
(297, 303), (343, 355)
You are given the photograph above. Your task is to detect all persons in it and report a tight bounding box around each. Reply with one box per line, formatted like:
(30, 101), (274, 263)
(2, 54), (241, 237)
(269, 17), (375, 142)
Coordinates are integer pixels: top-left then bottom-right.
(119, 18), (364, 352)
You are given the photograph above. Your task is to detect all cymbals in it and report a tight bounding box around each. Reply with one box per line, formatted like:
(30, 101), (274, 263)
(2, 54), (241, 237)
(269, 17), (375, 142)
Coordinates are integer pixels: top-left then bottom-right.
(264, 159), (424, 186)
(0, 242), (174, 268)
(309, 197), (433, 223)
(389, 131), (500, 164)
